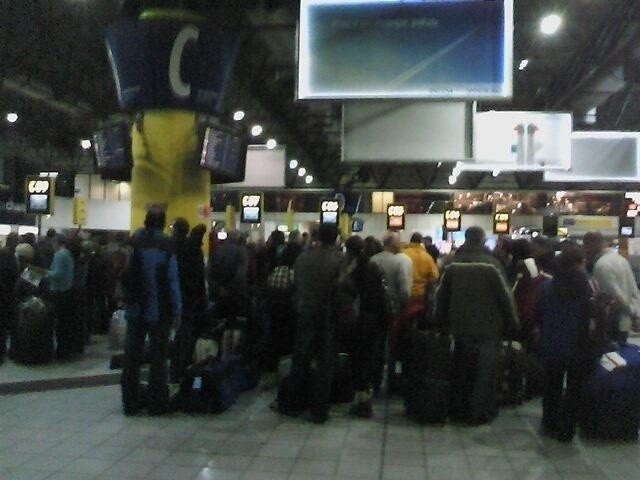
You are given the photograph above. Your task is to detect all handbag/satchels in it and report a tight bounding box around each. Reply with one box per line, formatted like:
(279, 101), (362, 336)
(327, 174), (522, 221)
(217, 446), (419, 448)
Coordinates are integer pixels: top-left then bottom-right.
(163, 324), (640, 444)
(8, 297), (54, 365)
